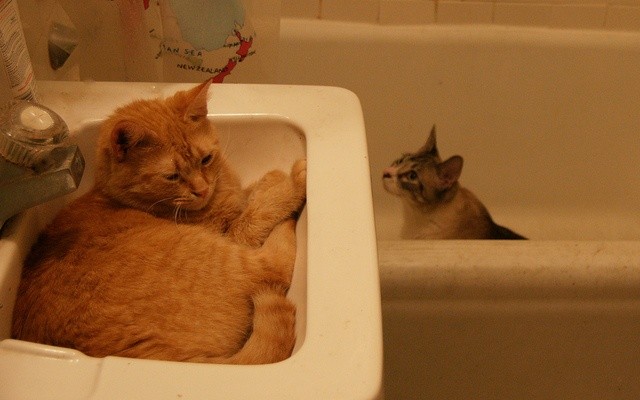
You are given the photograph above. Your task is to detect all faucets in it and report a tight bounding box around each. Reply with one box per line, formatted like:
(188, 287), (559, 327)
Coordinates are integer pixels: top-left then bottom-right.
(1, 102), (89, 193)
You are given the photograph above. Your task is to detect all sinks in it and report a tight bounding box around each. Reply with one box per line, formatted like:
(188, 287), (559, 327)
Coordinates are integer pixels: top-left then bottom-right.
(7, 113), (312, 369)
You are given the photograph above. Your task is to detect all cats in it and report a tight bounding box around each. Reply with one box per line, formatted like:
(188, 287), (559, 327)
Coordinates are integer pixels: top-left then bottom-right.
(11, 78), (306, 365)
(381, 124), (528, 240)
